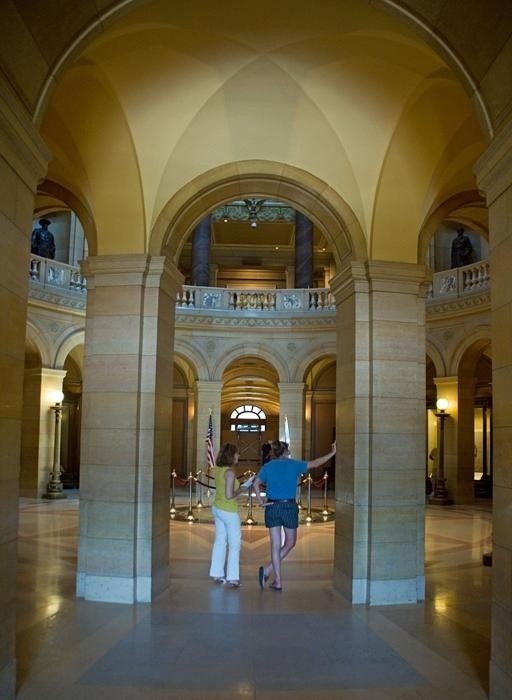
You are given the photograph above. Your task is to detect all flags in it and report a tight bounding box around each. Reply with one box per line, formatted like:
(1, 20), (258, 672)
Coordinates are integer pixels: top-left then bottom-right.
(204, 407), (217, 471)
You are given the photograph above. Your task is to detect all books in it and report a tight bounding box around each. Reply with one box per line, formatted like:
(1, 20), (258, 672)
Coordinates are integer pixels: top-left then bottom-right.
(240, 470), (257, 489)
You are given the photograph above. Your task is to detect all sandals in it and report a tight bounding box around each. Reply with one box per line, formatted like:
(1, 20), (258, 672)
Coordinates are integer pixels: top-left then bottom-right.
(211, 575), (243, 587)
(258, 565), (283, 592)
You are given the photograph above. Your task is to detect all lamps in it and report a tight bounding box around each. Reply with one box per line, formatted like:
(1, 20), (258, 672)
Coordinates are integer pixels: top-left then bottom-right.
(243, 198), (267, 228)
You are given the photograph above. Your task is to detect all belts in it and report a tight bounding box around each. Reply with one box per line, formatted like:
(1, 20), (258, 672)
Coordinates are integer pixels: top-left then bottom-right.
(267, 497), (297, 505)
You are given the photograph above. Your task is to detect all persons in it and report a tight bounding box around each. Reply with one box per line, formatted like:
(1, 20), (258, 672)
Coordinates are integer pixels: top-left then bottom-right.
(448, 226), (472, 268)
(29, 217), (55, 270)
(208, 441), (256, 588)
(252, 437), (337, 594)
(426, 448), (438, 497)
(259, 439), (273, 465)
